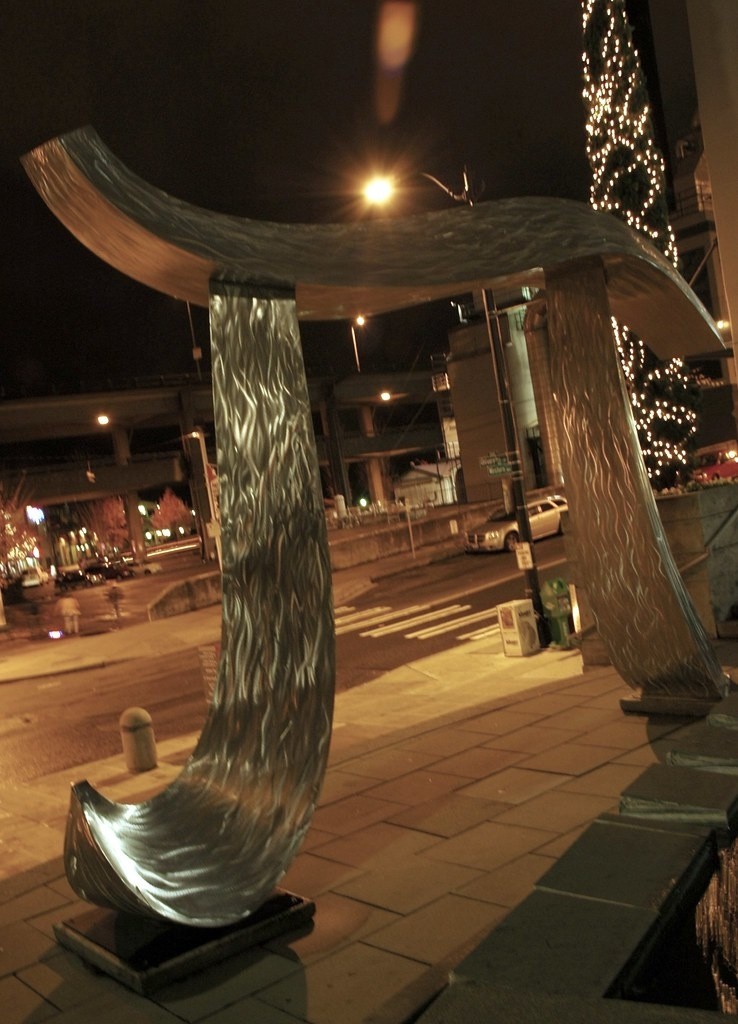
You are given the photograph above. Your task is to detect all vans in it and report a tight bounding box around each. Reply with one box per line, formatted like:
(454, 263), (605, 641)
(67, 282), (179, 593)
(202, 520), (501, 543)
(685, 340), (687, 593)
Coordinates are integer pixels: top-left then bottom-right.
(690, 440), (738, 487)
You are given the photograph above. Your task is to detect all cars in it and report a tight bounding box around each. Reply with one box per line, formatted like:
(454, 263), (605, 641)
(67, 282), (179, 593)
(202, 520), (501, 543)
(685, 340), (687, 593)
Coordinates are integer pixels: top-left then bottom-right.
(463, 496), (568, 554)
(20, 560), (162, 591)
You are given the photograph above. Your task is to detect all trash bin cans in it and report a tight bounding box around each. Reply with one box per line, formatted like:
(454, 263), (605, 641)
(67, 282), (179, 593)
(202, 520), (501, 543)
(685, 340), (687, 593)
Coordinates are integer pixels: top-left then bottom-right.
(540, 577), (574, 647)
(497, 598), (540, 658)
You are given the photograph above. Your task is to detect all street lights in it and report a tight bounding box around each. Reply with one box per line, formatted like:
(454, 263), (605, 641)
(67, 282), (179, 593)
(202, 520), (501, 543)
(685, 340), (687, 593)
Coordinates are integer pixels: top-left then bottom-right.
(351, 315), (365, 374)
(360, 169), (552, 650)
